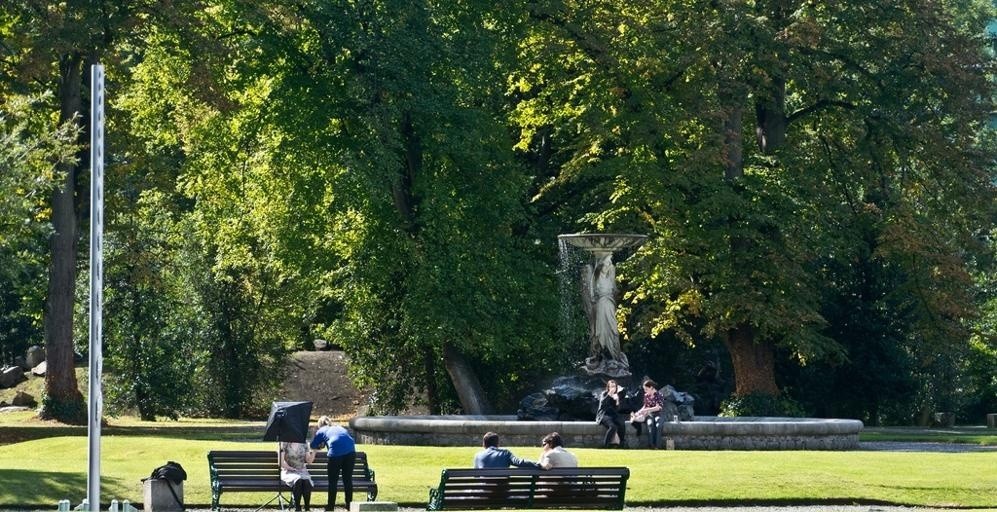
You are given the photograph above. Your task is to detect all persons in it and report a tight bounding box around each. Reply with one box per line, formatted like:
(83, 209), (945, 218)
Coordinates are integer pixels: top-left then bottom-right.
(308, 415), (356, 512)
(587, 256), (622, 361)
(470, 430), (545, 502)
(593, 379), (631, 450)
(628, 377), (666, 452)
(533, 430), (581, 498)
(277, 441), (317, 512)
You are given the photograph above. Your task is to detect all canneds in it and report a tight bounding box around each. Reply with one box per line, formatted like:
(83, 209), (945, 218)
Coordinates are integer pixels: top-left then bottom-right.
(673, 415), (678, 422)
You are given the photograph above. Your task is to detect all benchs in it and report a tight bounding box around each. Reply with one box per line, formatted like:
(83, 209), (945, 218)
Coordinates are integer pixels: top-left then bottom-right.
(206, 449), (377, 511)
(428, 466), (629, 511)
